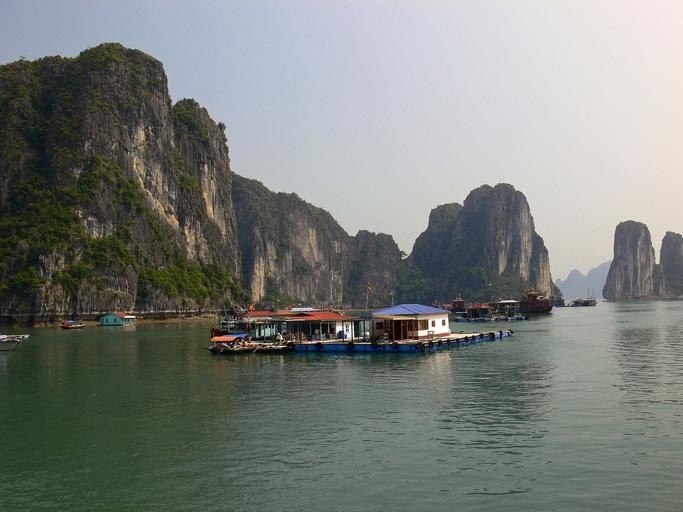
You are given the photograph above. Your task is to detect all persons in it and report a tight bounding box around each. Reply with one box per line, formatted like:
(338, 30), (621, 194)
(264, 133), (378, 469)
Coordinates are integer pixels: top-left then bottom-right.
(324, 330), (330, 340)
(313, 327), (319, 335)
(272, 332), (282, 345)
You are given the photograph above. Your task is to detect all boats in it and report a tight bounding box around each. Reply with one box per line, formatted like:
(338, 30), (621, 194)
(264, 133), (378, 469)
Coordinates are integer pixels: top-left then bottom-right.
(60, 320), (85, 329)
(206, 334), (287, 353)
(489, 290), (552, 313)
(0, 334), (29, 351)
(451, 300), (465, 312)
(551, 294), (596, 307)
